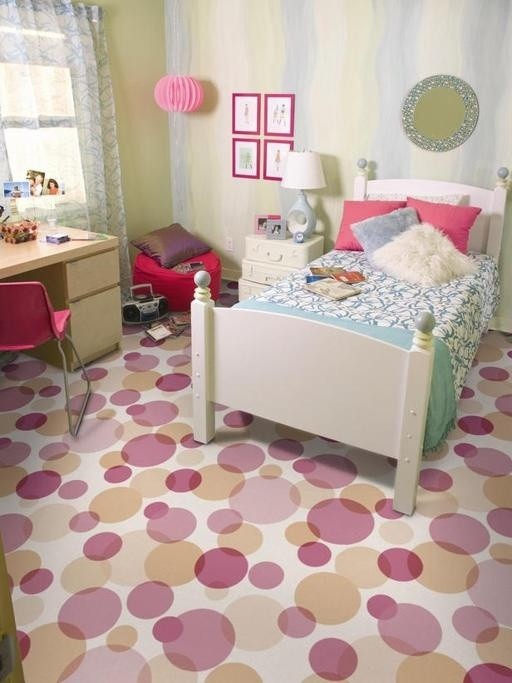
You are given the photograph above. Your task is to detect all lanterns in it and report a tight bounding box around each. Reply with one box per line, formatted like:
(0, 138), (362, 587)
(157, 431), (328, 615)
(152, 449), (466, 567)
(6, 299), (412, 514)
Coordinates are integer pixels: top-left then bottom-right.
(154, 76), (204, 113)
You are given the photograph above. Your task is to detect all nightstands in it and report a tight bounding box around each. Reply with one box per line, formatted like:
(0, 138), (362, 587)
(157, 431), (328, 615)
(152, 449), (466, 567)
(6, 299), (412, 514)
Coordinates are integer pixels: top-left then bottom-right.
(238, 234), (325, 302)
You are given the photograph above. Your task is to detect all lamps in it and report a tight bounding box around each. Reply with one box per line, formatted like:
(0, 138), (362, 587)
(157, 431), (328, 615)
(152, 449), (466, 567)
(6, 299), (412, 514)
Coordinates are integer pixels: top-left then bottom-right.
(279, 151), (327, 240)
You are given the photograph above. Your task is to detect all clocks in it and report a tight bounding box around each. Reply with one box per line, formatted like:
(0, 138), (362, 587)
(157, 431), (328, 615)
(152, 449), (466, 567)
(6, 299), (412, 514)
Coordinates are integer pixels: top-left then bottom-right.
(294, 231), (304, 243)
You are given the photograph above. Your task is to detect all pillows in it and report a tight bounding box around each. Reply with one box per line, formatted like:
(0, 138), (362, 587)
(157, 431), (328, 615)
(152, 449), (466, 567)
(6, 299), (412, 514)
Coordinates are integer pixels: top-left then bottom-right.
(129, 222), (213, 269)
(333, 192), (482, 289)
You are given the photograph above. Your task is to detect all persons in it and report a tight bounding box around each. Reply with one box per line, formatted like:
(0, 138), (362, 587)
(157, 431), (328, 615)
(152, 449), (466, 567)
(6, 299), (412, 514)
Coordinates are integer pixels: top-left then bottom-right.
(28, 173), (43, 196)
(259, 220), (267, 231)
(45, 178), (58, 195)
(11, 186), (23, 198)
(271, 225), (281, 234)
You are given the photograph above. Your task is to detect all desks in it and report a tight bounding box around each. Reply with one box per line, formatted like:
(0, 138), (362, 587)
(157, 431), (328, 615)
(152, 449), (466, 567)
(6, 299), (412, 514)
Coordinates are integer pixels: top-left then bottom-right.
(0, 225), (123, 372)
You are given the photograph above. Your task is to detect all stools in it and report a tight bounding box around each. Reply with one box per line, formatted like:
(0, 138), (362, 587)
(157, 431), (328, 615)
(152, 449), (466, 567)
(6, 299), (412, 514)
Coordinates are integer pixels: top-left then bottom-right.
(133, 251), (222, 310)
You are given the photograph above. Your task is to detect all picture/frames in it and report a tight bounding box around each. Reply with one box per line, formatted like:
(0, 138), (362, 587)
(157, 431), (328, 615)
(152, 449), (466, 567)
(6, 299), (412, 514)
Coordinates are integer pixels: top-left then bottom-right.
(231, 92), (295, 181)
(255, 214), (286, 240)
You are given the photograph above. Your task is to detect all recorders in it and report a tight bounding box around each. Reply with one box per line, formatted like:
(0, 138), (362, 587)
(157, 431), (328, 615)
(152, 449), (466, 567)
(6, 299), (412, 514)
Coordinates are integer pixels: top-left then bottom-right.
(121, 284), (169, 326)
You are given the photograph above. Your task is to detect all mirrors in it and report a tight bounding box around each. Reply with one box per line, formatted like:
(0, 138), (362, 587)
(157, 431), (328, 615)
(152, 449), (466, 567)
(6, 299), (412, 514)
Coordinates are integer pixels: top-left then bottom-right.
(401, 73), (479, 151)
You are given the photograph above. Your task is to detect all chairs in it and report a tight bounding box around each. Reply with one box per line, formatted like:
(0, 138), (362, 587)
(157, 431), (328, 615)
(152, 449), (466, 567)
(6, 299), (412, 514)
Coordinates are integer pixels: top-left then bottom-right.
(0, 281), (92, 438)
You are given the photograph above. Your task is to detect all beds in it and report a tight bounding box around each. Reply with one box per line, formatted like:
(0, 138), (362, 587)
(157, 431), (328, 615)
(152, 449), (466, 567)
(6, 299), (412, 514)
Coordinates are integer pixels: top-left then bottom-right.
(189, 158), (509, 517)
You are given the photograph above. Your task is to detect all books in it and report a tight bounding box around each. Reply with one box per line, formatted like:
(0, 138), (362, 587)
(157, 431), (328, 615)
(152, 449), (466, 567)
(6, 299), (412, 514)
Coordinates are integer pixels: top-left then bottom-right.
(303, 266), (367, 302)
(141, 308), (192, 344)
